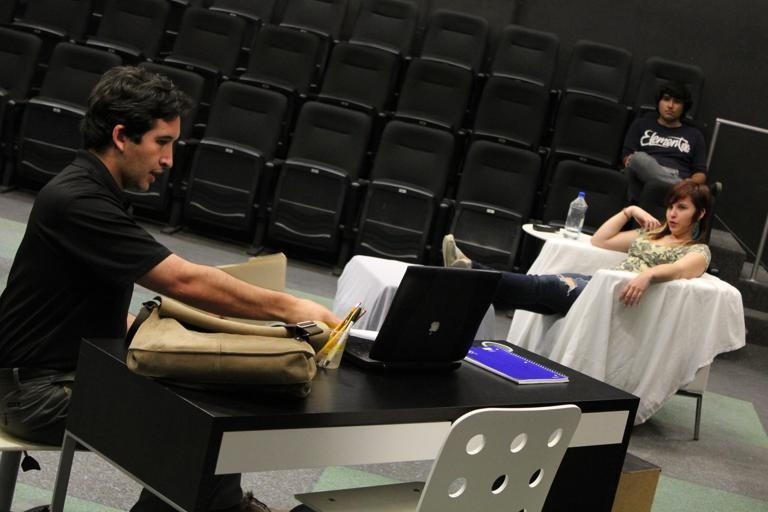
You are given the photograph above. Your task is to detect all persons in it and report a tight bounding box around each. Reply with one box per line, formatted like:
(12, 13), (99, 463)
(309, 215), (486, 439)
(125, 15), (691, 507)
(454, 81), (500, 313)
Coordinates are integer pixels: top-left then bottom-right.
(441, 172), (712, 309)
(0, 67), (341, 511)
(623, 81), (723, 217)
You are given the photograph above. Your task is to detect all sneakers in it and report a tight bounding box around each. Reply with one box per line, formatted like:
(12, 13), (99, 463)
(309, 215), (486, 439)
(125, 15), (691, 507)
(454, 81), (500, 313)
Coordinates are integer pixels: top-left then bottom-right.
(709, 182), (721, 196)
(442, 234), (471, 279)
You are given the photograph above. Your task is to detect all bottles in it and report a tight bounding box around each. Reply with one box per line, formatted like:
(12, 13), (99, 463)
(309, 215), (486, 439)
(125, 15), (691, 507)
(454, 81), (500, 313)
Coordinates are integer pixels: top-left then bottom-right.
(564, 192), (588, 241)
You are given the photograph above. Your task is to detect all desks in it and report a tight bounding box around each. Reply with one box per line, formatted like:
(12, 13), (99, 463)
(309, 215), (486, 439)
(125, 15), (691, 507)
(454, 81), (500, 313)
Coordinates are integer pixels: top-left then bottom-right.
(331, 254), (426, 330)
(49, 333), (642, 511)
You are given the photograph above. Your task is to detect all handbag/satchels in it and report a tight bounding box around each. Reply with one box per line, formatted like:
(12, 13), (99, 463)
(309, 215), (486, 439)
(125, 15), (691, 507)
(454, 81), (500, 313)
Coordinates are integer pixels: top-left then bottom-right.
(125, 252), (330, 395)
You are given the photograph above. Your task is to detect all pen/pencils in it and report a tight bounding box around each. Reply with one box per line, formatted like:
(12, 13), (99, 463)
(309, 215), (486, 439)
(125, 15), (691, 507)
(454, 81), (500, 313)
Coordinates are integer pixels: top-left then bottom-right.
(314, 300), (367, 369)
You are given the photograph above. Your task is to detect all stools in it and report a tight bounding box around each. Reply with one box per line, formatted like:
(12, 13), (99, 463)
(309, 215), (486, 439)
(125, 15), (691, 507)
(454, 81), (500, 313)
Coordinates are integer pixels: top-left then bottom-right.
(611, 453), (661, 511)
(0, 255), (94, 512)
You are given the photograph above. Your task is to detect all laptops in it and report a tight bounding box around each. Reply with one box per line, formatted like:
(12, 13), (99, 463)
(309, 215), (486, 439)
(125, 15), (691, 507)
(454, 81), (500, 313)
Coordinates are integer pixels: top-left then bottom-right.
(343, 264), (504, 373)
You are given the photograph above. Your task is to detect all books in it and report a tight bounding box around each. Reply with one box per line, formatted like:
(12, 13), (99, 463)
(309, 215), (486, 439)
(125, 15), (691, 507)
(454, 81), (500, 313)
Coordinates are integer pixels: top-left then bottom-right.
(464, 345), (571, 384)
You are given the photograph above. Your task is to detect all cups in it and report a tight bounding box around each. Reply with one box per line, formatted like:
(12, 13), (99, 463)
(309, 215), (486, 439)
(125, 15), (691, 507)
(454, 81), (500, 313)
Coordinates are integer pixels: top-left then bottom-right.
(312, 331), (350, 369)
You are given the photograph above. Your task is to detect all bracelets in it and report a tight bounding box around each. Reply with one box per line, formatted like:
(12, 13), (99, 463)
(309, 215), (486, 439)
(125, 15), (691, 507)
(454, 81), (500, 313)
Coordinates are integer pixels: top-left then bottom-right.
(622, 207), (631, 222)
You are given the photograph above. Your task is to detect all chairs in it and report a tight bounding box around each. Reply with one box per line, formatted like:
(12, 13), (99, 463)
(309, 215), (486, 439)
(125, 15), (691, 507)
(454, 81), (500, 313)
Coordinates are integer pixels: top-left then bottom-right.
(505, 234), (746, 440)
(295, 405), (582, 511)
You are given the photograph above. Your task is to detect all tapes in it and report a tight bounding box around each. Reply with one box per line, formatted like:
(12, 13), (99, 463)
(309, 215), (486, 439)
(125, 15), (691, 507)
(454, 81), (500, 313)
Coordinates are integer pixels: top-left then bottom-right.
(481, 341), (513, 353)
